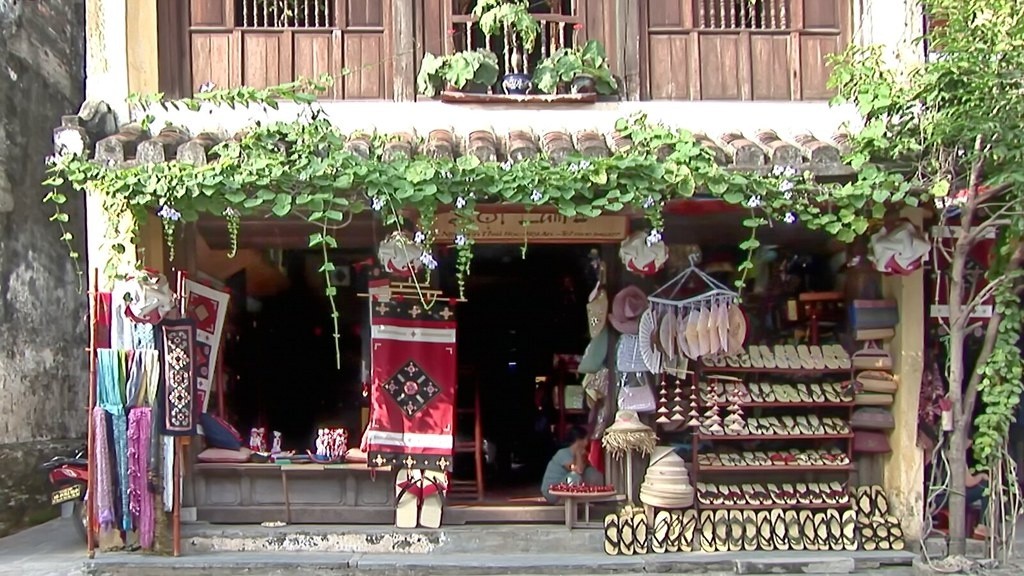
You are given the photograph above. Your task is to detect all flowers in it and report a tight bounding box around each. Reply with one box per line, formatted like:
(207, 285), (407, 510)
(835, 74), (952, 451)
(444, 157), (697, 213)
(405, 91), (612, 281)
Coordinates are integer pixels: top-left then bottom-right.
(533, 23), (618, 95)
(417, 31), (499, 97)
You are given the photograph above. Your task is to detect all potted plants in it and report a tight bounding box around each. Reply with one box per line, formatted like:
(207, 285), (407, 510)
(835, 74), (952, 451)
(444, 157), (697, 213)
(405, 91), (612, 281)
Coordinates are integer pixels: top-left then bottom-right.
(471, 0), (540, 95)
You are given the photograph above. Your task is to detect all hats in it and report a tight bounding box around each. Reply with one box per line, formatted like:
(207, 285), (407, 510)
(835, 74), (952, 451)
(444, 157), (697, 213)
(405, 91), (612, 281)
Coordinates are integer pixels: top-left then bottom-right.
(608, 285), (649, 335)
(586, 290), (608, 338)
(605, 410), (654, 433)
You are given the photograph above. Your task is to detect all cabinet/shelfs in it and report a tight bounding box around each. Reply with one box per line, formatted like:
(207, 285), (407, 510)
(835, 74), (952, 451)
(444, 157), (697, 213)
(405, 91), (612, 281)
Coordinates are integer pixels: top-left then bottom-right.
(558, 310), (855, 512)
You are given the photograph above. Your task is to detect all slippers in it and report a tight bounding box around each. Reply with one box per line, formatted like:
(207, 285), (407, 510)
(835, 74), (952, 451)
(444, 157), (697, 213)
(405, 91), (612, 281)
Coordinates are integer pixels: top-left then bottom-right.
(395, 467), (422, 528)
(850, 485), (905, 551)
(696, 481), (848, 505)
(698, 414), (849, 435)
(697, 446), (850, 466)
(701, 344), (852, 369)
(419, 469), (448, 528)
(602, 508), (859, 556)
(698, 381), (853, 403)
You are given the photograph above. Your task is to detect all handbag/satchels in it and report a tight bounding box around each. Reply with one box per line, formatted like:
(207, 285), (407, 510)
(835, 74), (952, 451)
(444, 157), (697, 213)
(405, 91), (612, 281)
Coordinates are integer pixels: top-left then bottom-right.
(849, 298), (900, 453)
(616, 333), (650, 373)
(617, 374), (656, 415)
(576, 327), (609, 375)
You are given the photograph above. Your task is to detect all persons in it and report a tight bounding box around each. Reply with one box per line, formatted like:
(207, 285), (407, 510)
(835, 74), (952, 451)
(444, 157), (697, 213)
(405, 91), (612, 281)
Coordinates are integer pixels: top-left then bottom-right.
(541, 423), (593, 505)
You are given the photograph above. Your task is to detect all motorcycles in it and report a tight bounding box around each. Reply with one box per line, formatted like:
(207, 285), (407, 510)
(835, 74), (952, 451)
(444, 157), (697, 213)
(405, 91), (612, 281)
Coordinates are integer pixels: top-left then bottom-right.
(41, 442), (99, 548)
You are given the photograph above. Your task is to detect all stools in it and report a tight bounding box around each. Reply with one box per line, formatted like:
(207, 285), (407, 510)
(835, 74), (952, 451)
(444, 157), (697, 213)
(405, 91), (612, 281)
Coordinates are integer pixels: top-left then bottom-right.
(565, 495), (626, 530)
(932, 506), (980, 538)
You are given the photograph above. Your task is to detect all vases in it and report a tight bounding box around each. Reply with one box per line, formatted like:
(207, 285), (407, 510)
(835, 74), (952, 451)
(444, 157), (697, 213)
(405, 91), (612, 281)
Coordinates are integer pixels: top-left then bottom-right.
(445, 79), (487, 93)
(571, 74), (594, 93)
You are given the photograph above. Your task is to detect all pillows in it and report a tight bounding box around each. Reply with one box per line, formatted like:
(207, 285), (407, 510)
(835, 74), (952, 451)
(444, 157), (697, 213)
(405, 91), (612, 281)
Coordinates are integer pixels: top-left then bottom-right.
(345, 448), (368, 463)
(200, 413), (241, 450)
(198, 447), (250, 463)
